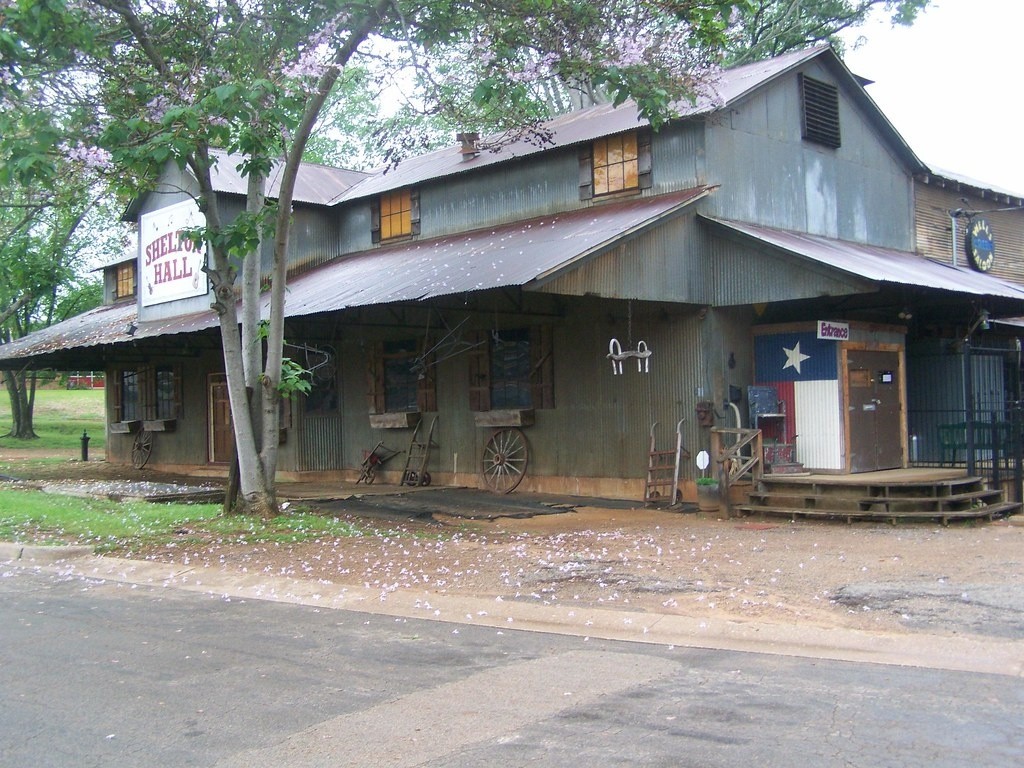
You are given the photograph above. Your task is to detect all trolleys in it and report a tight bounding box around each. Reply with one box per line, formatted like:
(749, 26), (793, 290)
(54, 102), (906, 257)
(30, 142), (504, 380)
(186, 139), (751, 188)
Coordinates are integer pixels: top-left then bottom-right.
(400, 413), (440, 490)
(355, 440), (406, 484)
(642, 418), (690, 507)
